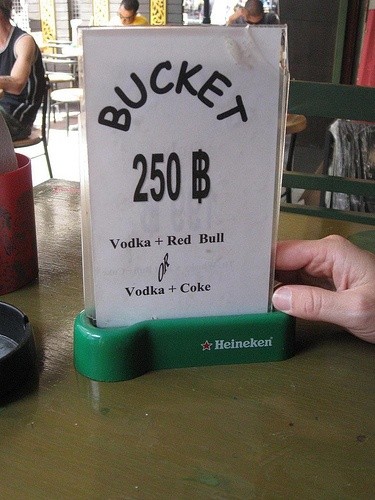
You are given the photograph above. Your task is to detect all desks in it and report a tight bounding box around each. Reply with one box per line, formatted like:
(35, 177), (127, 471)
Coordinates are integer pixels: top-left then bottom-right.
(0, 178), (375, 500)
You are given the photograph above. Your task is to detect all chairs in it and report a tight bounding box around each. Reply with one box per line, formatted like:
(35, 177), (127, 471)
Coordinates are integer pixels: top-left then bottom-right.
(12, 78), (53, 178)
(281, 112), (307, 204)
(43, 58), (78, 88)
(50, 88), (83, 133)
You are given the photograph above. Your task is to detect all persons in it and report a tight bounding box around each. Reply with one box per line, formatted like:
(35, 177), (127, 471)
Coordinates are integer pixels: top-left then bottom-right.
(0, 0), (45, 142)
(269, 234), (374, 343)
(107, 1), (149, 27)
(226, 0), (280, 25)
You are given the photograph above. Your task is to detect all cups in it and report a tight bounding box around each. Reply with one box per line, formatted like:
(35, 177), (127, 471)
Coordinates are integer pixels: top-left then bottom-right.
(0, 154), (40, 295)
